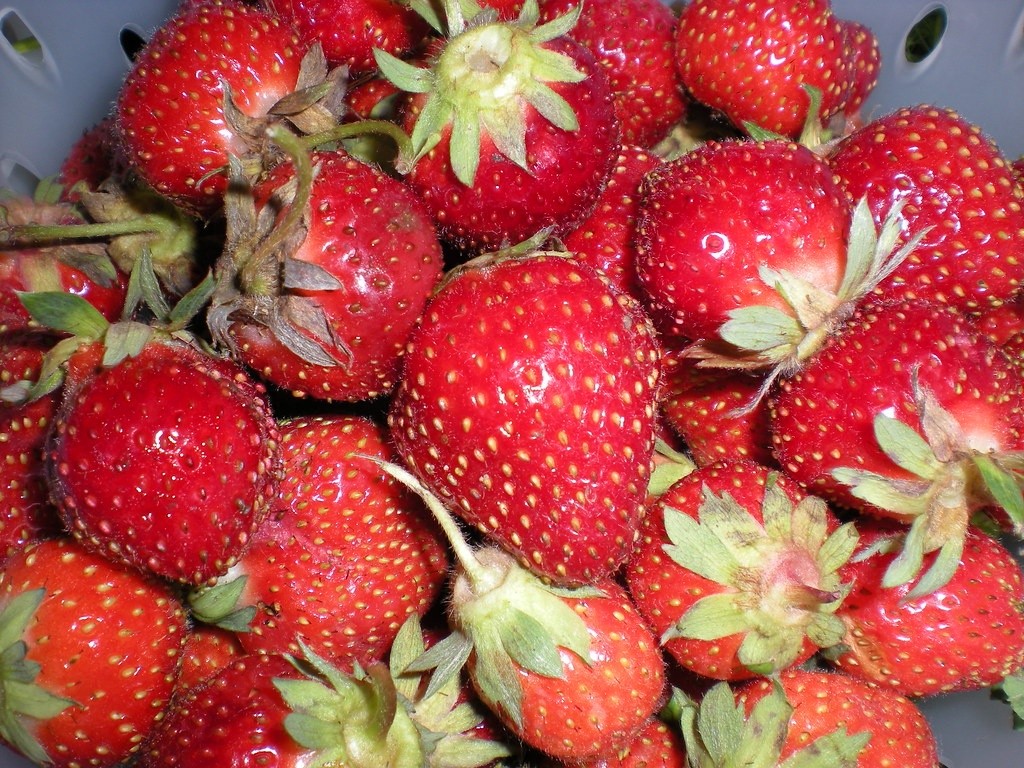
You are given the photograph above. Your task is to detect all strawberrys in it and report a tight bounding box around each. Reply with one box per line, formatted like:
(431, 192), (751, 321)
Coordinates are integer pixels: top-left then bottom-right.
(0, 0), (1024, 768)
